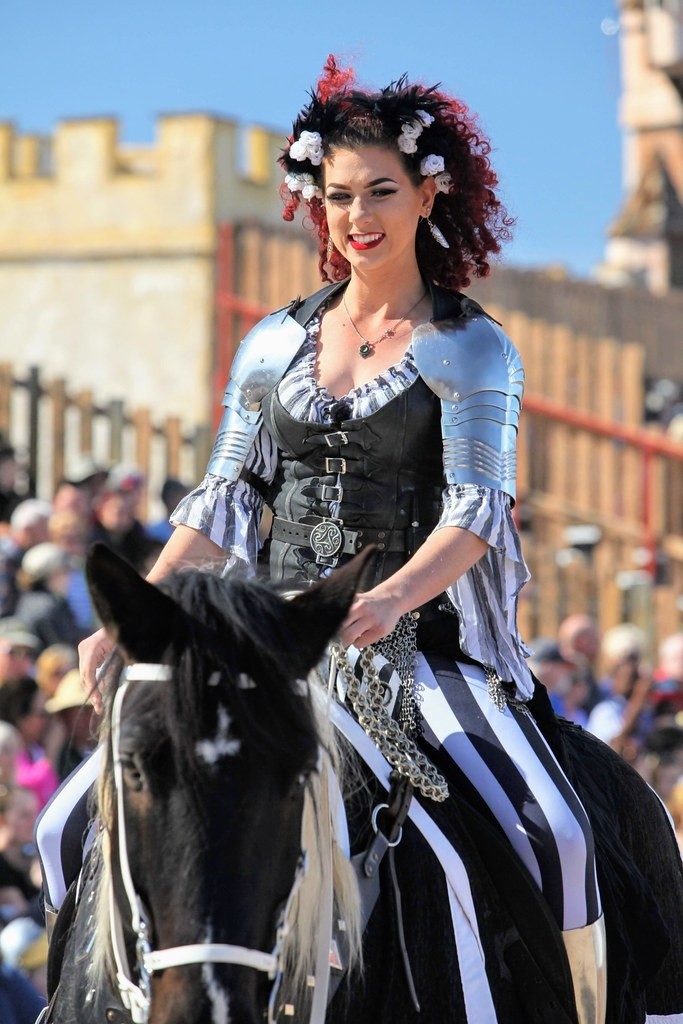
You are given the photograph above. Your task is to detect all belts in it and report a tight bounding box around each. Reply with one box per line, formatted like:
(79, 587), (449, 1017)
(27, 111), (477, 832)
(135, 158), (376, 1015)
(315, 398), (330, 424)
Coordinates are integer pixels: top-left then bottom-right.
(269, 515), (423, 557)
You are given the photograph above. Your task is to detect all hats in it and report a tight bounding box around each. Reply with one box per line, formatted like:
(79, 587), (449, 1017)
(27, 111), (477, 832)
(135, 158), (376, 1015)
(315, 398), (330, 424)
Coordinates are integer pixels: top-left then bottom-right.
(526, 639), (582, 670)
(1, 619), (42, 652)
(20, 541), (88, 581)
(60, 456), (108, 489)
(45, 667), (95, 713)
(1, 918), (49, 971)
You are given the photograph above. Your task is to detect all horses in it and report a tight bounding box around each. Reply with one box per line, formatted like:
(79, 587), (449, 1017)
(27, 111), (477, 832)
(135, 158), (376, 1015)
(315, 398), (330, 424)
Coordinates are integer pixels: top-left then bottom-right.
(34, 541), (683, 1024)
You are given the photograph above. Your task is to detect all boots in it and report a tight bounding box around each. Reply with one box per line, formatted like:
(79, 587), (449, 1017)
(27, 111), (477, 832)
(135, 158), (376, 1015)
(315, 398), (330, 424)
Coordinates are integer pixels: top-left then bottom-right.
(561, 911), (607, 1024)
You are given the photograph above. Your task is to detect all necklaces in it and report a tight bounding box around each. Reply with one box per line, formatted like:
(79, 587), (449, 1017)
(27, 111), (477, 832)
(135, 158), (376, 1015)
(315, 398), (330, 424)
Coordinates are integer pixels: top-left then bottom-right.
(335, 278), (429, 357)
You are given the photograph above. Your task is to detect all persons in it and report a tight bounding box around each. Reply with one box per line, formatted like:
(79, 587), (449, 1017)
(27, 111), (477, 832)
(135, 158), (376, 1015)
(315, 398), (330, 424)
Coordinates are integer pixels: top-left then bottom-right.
(34, 80), (610, 1023)
(0, 435), (683, 1024)
